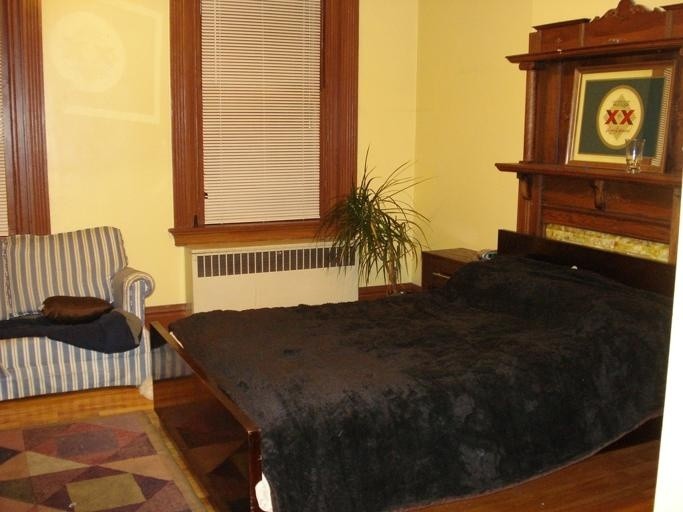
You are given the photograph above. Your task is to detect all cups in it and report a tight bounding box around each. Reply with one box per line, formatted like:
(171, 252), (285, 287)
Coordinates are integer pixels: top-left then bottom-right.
(625, 138), (646, 174)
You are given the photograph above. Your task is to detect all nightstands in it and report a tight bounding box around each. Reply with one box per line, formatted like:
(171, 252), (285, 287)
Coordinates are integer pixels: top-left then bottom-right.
(422, 246), (480, 287)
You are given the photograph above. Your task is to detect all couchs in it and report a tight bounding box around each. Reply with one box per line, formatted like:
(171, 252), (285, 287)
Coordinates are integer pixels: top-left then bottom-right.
(0, 225), (154, 403)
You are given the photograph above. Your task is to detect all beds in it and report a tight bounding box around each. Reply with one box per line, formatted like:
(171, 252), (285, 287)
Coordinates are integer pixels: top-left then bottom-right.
(149, 226), (674, 512)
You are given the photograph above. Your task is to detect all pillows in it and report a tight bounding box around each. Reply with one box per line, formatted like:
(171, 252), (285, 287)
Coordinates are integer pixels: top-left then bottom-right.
(41, 295), (115, 326)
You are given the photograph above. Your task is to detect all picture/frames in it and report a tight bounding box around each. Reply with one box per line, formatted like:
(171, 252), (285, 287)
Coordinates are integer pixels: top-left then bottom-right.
(563, 58), (676, 176)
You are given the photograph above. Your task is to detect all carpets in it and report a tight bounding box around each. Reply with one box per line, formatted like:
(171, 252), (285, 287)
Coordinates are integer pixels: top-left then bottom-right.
(0, 409), (208, 512)
